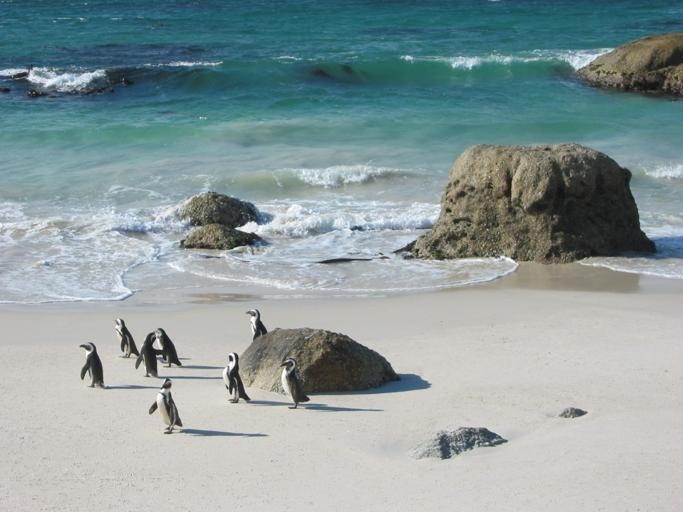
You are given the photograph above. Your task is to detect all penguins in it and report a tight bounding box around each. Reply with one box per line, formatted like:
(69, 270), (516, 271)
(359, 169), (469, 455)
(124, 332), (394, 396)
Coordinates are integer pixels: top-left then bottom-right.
(135, 331), (163, 378)
(278, 356), (310, 410)
(79, 341), (106, 389)
(245, 308), (268, 343)
(154, 327), (183, 367)
(221, 351), (251, 404)
(113, 317), (139, 358)
(148, 378), (184, 435)
